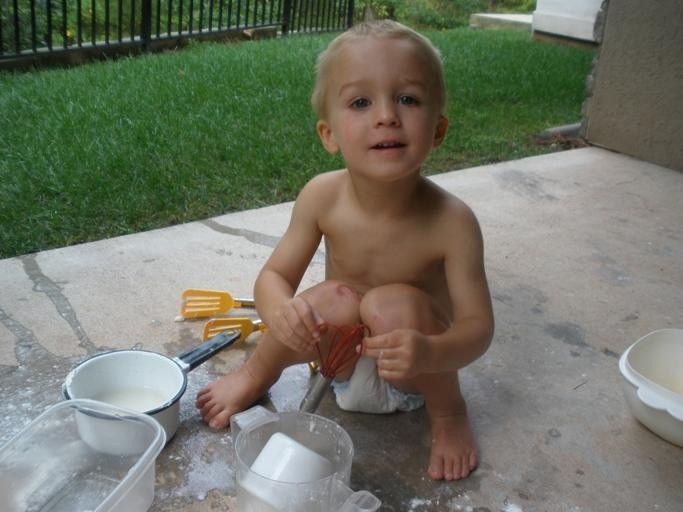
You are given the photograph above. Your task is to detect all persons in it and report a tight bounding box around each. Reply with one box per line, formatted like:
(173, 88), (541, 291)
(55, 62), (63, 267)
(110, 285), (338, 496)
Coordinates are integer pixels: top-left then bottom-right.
(195, 20), (494, 481)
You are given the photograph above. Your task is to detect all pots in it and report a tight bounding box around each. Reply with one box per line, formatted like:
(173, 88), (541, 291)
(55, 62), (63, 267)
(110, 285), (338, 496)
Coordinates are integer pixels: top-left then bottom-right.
(63, 328), (241, 457)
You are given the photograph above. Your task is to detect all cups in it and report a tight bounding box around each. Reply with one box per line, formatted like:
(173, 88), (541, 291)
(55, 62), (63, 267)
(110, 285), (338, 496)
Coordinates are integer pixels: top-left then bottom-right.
(230, 404), (382, 512)
(241, 432), (333, 511)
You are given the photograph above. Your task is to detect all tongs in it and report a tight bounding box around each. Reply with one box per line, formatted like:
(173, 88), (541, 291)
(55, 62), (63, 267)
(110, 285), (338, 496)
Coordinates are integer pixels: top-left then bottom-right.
(180, 288), (263, 347)
